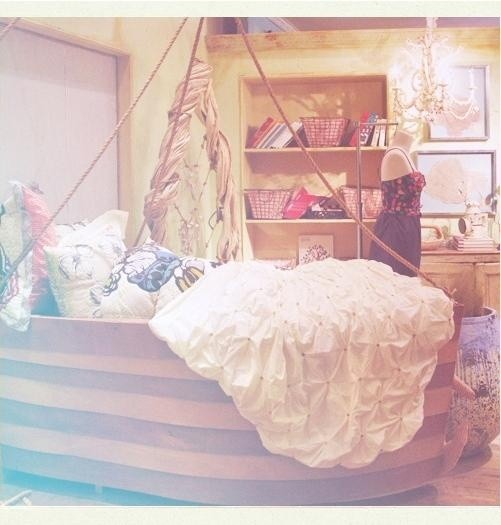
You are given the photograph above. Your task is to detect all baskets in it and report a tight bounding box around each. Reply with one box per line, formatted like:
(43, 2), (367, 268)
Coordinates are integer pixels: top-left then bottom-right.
(299, 116), (350, 148)
(421, 224), (443, 250)
(244, 189), (293, 219)
(337, 185), (382, 219)
(307, 209), (343, 220)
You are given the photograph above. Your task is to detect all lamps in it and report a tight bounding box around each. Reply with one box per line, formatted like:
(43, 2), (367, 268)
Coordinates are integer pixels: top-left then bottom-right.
(392, 12), (482, 128)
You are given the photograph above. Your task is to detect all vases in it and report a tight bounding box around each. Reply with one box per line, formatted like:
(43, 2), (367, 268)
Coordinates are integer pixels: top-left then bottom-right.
(452, 302), (499, 464)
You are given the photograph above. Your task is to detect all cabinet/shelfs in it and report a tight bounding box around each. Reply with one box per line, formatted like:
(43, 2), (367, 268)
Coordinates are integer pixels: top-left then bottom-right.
(239, 73), (393, 266)
(418, 248), (500, 357)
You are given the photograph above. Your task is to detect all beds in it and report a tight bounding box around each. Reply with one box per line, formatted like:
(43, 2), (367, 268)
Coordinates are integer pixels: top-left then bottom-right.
(0, 255), (464, 505)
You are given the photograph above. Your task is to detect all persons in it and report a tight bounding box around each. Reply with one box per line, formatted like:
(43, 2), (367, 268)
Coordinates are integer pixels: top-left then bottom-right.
(367, 132), (427, 275)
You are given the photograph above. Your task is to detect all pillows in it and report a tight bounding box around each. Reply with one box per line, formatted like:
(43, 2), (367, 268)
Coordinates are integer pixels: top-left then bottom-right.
(0, 181), (229, 333)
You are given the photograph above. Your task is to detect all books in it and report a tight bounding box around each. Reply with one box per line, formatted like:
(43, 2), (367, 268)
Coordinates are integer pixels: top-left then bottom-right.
(350, 112), (386, 148)
(282, 185), (327, 219)
(451, 234), (495, 254)
(248, 116), (304, 148)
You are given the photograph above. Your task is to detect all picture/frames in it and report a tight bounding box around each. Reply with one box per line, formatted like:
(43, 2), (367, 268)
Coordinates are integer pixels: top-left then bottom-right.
(411, 149), (497, 218)
